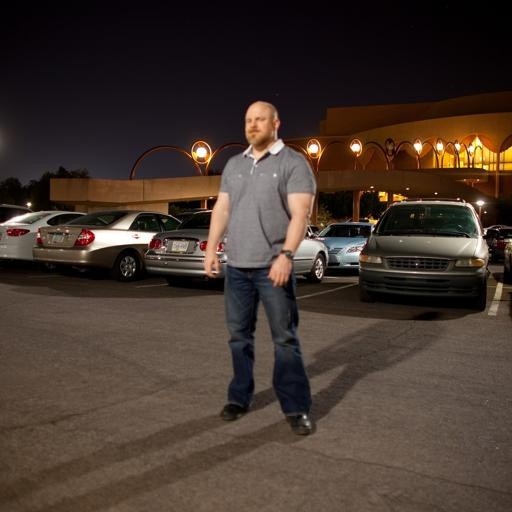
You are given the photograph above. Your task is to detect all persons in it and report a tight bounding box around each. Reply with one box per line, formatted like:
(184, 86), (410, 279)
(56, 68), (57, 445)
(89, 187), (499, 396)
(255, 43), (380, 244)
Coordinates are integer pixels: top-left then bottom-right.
(202, 101), (318, 435)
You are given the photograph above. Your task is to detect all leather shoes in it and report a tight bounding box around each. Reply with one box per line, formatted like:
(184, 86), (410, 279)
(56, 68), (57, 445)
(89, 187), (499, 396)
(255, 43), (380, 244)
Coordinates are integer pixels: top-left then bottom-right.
(285, 410), (312, 436)
(220, 398), (255, 421)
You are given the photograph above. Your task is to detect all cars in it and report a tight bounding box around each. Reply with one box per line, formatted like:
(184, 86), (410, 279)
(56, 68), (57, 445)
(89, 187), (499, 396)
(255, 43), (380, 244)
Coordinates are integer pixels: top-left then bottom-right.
(357, 198), (489, 310)
(318, 221), (376, 276)
(0, 210), (88, 271)
(31, 208), (182, 283)
(143, 208), (330, 291)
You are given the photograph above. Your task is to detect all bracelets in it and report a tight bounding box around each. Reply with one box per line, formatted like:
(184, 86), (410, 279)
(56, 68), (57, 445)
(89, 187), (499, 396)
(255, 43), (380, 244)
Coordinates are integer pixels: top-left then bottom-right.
(279, 249), (294, 260)
